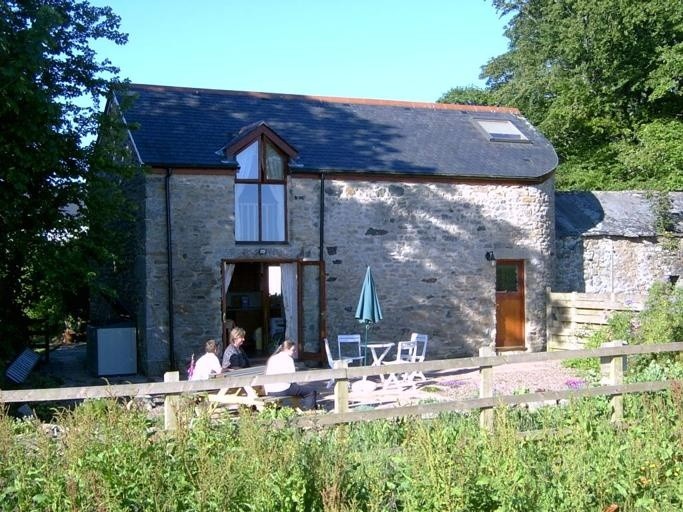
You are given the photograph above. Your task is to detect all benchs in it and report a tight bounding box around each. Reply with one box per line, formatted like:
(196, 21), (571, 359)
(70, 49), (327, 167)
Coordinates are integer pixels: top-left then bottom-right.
(205, 393), (306, 417)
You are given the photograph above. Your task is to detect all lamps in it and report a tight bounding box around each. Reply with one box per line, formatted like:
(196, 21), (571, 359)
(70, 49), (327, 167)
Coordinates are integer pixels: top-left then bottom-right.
(484, 250), (497, 269)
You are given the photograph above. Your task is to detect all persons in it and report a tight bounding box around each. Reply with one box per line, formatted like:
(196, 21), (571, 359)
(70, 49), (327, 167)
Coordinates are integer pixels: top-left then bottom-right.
(189, 340), (243, 396)
(262, 339), (332, 412)
(222, 327), (259, 393)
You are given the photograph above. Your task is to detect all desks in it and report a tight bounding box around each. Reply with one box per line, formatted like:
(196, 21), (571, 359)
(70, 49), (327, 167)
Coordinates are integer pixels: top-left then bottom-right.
(207, 365), (300, 412)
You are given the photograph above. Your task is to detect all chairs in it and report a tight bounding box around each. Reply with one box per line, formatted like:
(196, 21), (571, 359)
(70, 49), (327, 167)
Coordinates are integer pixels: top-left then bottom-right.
(320, 330), (429, 406)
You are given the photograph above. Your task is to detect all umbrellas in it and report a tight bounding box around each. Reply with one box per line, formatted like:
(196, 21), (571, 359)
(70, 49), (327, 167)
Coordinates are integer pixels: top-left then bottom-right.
(354, 265), (384, 367)
